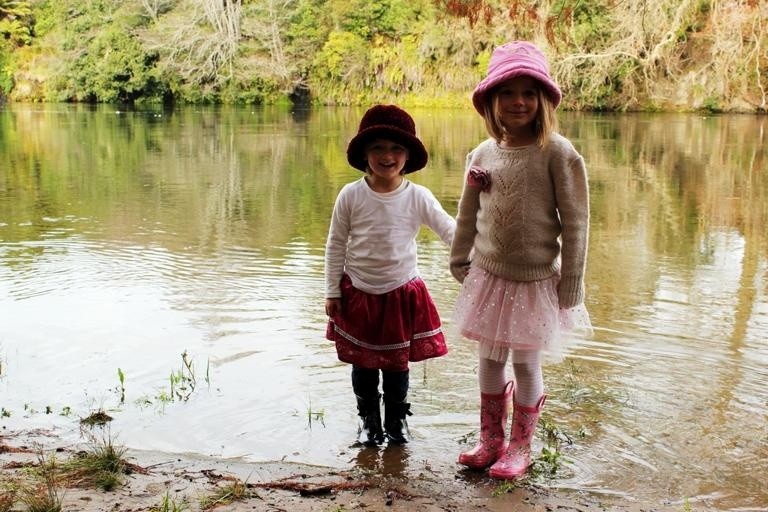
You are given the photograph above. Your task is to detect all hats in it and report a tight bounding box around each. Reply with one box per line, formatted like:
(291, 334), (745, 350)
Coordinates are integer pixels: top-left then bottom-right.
(473, 41), (562, 116)
(347, 106), (428, 175)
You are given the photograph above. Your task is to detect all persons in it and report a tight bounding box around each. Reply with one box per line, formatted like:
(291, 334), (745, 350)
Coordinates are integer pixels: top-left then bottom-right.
(324, 105), (457, 448)
(447, 42), (590, 478)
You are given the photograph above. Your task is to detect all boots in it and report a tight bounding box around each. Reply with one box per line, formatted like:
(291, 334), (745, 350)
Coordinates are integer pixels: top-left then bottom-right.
(459, 381), (513, 468)
(352, 381), (413, 448)
(488, 390), (546, 479)
